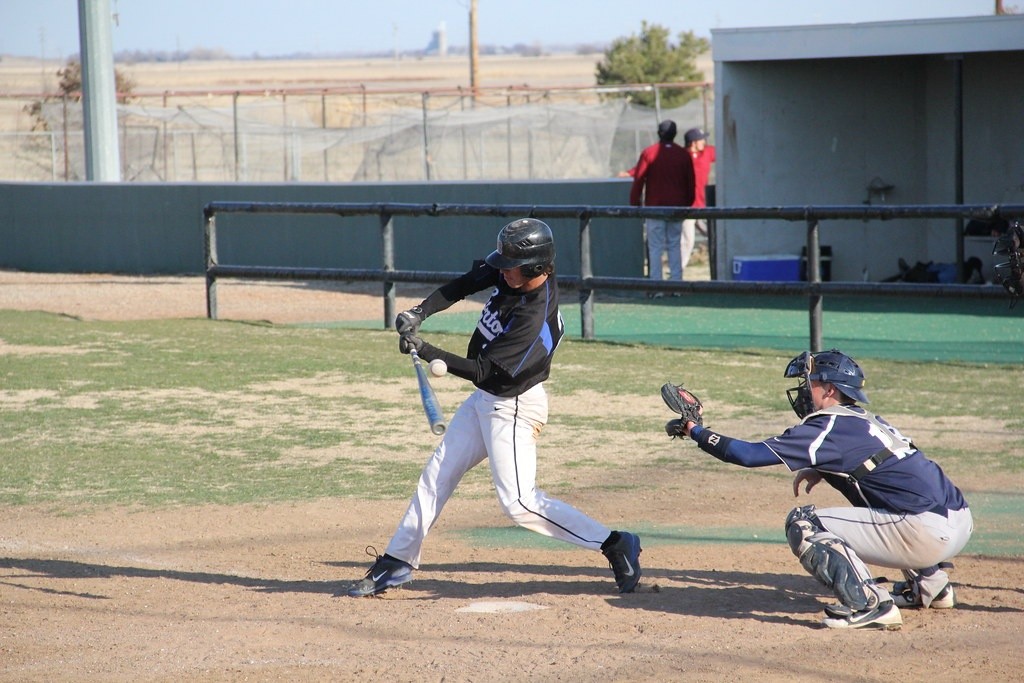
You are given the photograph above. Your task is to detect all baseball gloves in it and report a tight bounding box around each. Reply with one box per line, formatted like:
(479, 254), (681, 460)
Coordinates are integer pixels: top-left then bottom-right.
(661, 383), (703, 436)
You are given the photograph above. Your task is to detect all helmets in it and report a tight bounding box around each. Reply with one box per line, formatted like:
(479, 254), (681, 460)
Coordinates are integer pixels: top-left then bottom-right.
(804, 347), (870, 405)
(485, 217), (556, 278)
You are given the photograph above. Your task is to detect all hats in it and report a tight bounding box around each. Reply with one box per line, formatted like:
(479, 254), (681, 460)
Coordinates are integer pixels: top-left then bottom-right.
(685, 128), (710, 142)
(658, 120), (676, 138)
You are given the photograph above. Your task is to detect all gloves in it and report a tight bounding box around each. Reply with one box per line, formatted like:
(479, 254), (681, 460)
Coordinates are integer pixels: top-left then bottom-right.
(399, 332), (424, 355)
(395, 304), (427, 336)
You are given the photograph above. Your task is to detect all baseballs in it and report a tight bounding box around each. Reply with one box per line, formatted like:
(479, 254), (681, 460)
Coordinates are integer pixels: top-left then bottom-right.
(428, 359), (447, 377)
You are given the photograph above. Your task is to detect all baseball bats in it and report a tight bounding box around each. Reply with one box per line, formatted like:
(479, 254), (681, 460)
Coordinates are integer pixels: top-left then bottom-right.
(409, 343), (446, 436)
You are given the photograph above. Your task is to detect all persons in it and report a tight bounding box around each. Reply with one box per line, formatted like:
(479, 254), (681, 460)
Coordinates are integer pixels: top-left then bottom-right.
(660, 349), (974, 629)
(619, 127), (716, 267)
(629, 120), (696, 300)
(345, 217), (643, 597)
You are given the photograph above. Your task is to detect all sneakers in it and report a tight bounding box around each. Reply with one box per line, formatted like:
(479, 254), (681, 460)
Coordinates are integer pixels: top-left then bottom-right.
(822, 600), (904, 631)
(602, 531), (644, 593)
(889, 583), (955, 608)
(346, 544), (413, 597)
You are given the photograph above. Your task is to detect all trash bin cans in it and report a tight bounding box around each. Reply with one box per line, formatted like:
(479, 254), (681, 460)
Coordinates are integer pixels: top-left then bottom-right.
(801, 245), (834, 281)
(962, 220), (1008, 284)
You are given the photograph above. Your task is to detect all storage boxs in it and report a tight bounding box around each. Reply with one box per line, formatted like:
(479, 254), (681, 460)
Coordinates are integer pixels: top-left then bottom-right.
(733, 252), (802, 283)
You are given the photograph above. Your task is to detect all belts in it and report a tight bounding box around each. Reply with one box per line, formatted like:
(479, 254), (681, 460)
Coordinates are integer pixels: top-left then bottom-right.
(929, 505), (950, 518)
